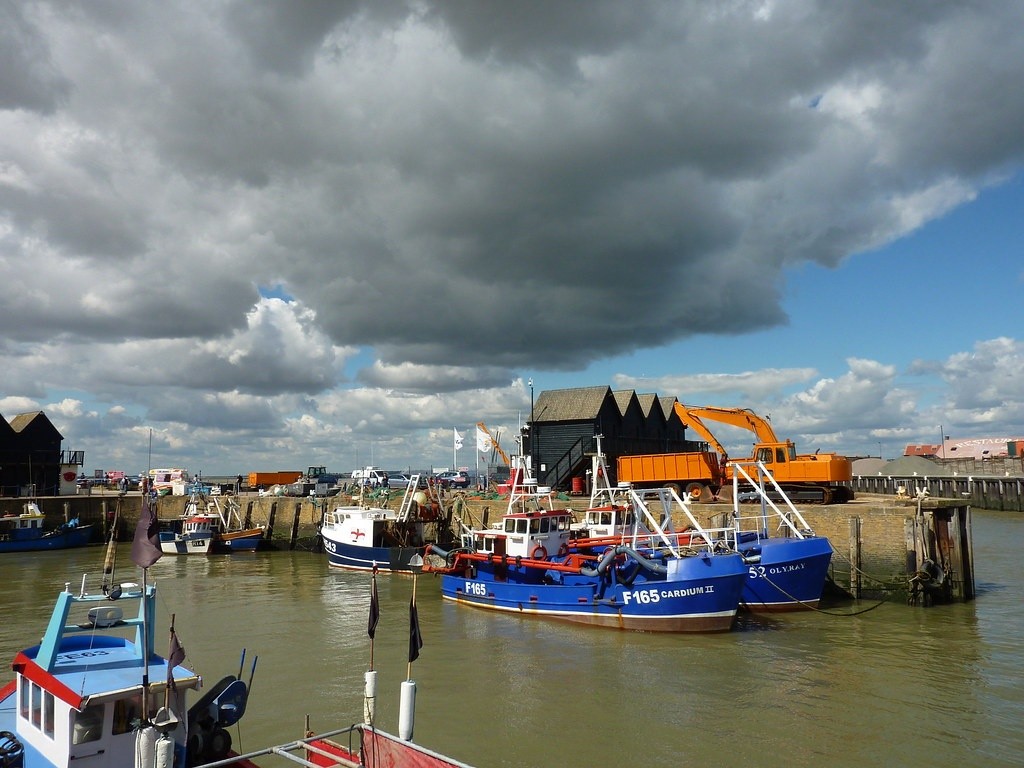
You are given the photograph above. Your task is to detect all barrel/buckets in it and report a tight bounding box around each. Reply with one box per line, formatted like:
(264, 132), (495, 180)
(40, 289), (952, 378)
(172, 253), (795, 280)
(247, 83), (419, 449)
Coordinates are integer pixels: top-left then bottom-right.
(573, 478), (582, 492)
(510, 468), (523, 491)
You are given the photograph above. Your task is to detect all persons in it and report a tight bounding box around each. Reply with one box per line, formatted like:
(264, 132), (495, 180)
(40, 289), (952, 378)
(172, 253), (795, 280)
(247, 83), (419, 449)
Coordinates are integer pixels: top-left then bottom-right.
(192, 474), (198, 484)
(419, 472), (435, 486)
(81, 472), (153, 495)
(365, 473), (388, 493)
(236, 474), (243, 495)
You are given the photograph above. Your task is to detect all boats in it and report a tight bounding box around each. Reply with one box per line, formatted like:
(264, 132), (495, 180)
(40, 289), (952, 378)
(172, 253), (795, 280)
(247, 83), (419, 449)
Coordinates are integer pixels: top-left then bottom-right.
(316, 432), (835, 632)
(0, 457), (96, 553)
(0, 474), (263, 768)
(158, 488), (265, 554)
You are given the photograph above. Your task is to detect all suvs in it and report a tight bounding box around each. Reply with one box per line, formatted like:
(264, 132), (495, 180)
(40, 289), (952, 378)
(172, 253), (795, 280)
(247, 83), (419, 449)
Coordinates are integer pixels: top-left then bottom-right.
(434, 471), (471, 489)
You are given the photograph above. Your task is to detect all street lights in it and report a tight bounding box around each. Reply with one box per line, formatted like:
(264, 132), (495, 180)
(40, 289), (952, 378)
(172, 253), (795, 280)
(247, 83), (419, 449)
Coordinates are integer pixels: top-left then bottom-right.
(527, 379), (535, 479)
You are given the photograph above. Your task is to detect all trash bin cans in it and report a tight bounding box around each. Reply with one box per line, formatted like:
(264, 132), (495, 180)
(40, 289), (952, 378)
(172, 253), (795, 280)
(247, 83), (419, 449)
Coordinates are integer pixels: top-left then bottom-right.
(159, 488), (170, 496)
(187, 486), (211, 496)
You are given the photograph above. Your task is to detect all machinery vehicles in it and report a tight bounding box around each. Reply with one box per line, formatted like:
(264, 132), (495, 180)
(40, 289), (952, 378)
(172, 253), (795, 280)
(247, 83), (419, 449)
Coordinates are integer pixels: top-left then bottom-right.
(672, 400), (856, 505)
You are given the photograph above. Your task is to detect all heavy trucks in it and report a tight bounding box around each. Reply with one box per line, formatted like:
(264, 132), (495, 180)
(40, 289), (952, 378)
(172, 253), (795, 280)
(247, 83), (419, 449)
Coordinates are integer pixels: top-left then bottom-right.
(247, 466), (339, 492)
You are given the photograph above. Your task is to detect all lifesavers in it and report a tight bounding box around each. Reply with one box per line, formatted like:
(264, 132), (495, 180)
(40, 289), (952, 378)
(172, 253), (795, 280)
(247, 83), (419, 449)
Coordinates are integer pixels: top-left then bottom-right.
(530, 545), (547, 562)
(558, 543), (569, 556)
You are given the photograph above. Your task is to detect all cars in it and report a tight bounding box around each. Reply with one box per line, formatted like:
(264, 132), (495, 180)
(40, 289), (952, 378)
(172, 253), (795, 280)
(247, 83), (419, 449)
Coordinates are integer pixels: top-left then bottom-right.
(387, 474), (428, 492)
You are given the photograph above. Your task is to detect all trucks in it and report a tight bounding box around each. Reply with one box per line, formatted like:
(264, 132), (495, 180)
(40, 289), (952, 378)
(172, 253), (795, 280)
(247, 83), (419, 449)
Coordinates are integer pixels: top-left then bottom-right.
(351, 469), (388, 489)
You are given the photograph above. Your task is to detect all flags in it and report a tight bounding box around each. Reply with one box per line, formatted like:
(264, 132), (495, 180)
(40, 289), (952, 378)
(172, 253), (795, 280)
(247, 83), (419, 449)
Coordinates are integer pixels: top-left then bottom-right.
(454, 428), (462, 450)
(477, 427), (492, 452)
